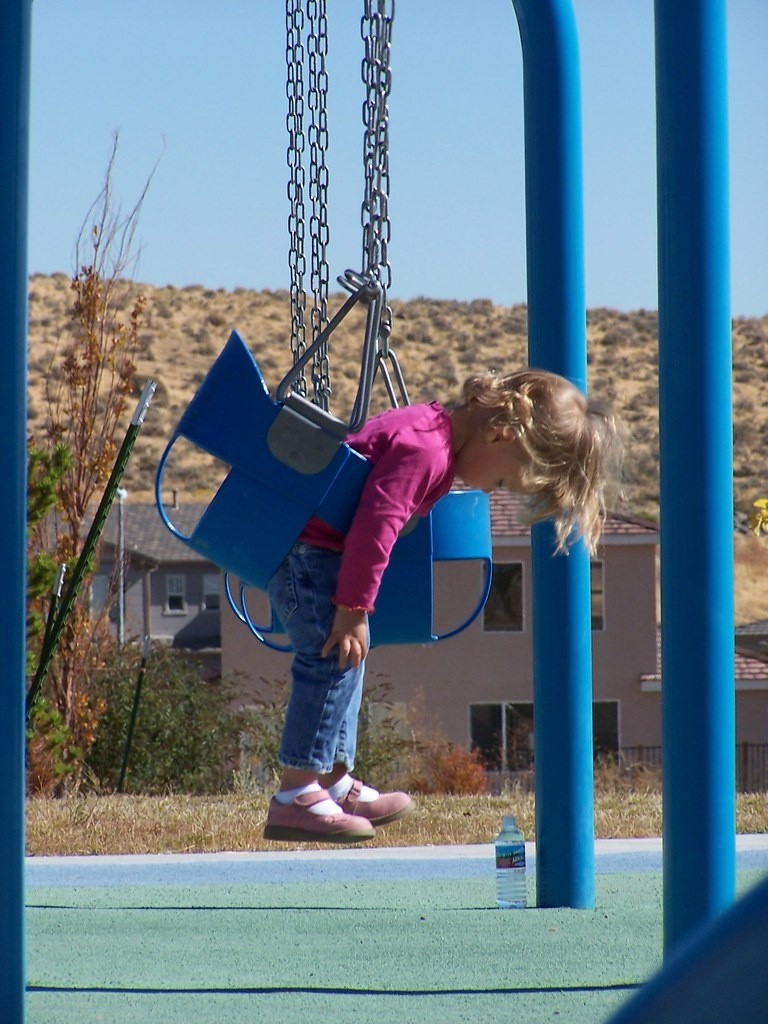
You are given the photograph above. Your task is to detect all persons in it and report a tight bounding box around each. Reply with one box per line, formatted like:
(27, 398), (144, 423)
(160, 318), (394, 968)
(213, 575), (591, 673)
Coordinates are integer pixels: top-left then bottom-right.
(265, 365), (598, 846)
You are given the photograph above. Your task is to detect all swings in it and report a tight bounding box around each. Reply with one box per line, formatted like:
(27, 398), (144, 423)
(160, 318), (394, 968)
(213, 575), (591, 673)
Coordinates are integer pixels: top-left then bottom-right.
(153, 1), (500, 661)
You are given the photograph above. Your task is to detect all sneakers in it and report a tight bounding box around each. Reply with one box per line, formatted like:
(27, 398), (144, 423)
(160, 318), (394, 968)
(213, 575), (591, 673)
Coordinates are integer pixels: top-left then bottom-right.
(334, 778), (416, 826)
(263, 789), (376, 843)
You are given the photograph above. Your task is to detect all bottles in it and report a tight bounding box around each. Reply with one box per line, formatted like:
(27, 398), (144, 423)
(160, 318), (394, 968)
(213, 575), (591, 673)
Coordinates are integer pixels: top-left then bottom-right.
(494, 815), (529, 909)
